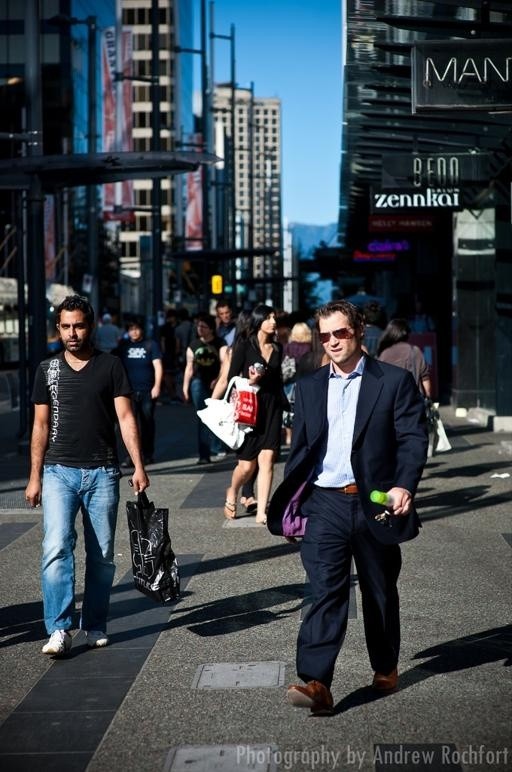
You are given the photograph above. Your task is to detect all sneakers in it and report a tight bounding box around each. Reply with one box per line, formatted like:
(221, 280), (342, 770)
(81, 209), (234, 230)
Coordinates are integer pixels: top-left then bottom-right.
(85, 628), (109, 647)
(41, 631), (74, 658)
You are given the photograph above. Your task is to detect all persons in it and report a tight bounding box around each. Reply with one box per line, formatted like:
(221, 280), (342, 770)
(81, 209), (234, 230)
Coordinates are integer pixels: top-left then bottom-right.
(95, 313), (119, 354)
(267, 300), (429, 712)
(211, 309), (258, 512)
(224, 305), (292, 524)
(111, 323), (162, 468)
(183, 315), (229, 465)
(215, 304), (235, 346)
(283, 321), (314, 445)
(378, 318), (440, 460)
(26, 295), (150, 655)
(162, 307), (188, 384)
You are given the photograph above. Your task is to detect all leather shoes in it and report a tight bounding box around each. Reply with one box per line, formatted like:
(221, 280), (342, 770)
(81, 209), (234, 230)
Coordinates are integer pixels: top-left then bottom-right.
(370, 670), (400, 692)
(284, 678), (334, 717)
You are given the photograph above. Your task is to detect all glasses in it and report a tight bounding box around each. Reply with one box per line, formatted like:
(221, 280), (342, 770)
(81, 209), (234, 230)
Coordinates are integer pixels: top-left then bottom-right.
(316, 327), (357, 342)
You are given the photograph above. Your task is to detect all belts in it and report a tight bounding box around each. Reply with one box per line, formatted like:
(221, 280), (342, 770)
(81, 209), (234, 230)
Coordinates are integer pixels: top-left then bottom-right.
(334, 482), (358, 494)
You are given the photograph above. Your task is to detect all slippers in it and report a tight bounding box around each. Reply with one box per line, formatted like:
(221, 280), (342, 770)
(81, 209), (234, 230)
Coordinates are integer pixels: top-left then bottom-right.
(224, 491), (238, 522)
(257, 516), (267, 524)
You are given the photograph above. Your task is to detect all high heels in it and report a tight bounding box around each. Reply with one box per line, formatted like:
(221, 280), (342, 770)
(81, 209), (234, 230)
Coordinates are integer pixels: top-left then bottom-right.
(240, 497), (257, 514)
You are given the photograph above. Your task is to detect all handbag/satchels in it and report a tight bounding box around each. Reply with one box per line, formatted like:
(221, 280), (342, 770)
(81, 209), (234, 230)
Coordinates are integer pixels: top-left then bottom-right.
(430, 402), (452, 453)
(125, 495), (181, 606)
(425, 407), (436, 457)
(197, 376), (254, 450)
(232, 374), (259, 426)
